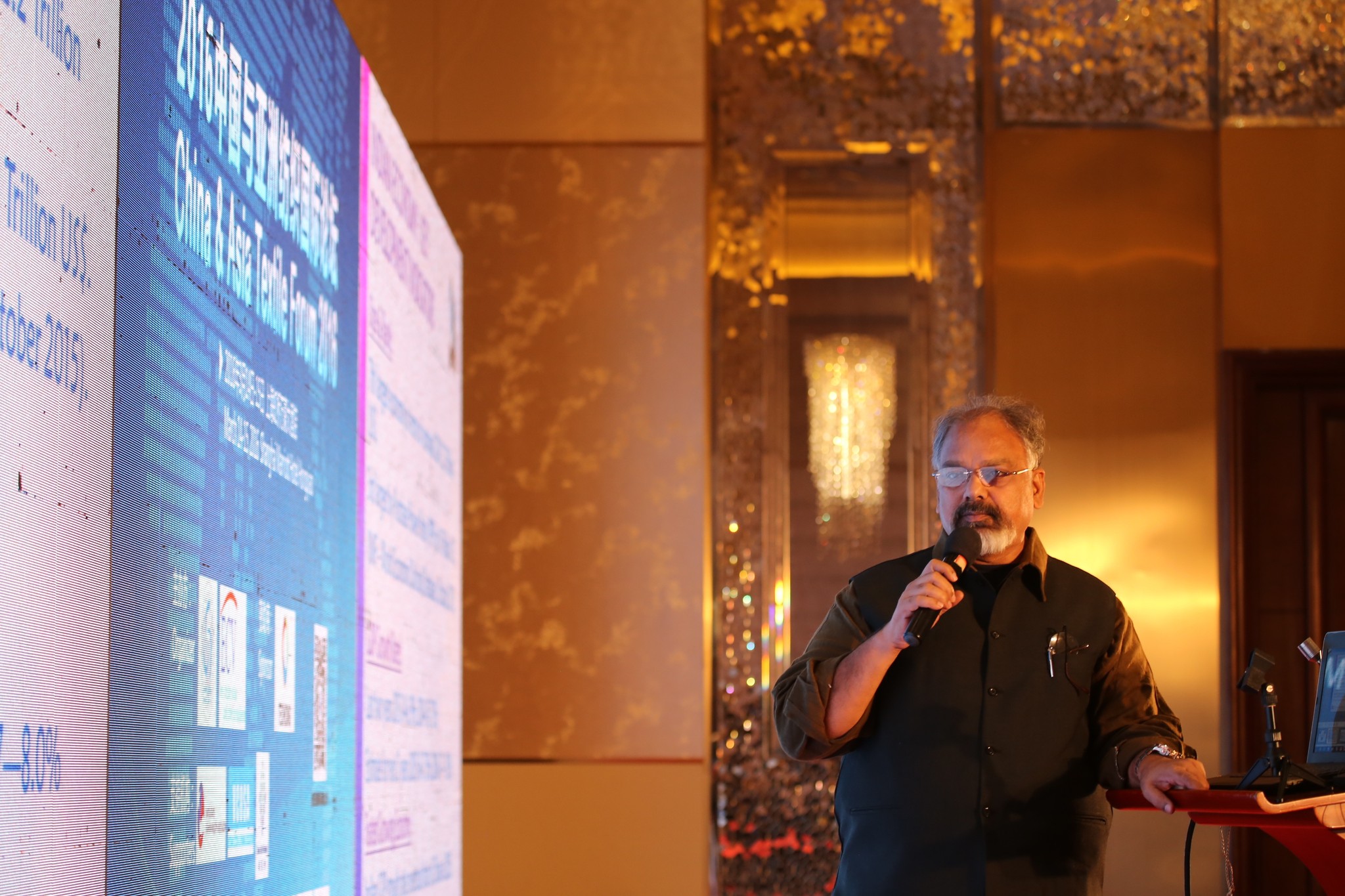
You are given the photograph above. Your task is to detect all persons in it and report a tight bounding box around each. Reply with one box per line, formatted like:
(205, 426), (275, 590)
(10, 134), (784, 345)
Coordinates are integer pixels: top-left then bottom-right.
(773, 392), (1211, 896)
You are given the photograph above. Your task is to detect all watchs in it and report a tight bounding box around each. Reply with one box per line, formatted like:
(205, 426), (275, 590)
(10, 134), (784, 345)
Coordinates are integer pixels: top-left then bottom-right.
(1135, 743), (1183, 777)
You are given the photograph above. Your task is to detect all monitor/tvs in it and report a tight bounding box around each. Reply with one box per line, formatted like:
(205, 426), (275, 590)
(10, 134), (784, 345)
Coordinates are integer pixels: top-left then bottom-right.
(1307, 630), (1345, 764)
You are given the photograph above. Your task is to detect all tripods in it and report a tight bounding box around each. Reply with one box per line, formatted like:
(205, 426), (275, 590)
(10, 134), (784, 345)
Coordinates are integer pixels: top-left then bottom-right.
(1233, 650), (1334, 804)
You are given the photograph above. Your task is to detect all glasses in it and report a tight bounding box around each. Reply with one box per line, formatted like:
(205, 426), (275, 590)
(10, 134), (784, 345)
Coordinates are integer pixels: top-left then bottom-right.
(932, 467), (1035, 488)
(1047, 631), (1091, 695)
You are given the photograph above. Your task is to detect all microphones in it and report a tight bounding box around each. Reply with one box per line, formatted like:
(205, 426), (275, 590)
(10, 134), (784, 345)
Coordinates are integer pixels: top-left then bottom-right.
(904, 527), (982, 648)
